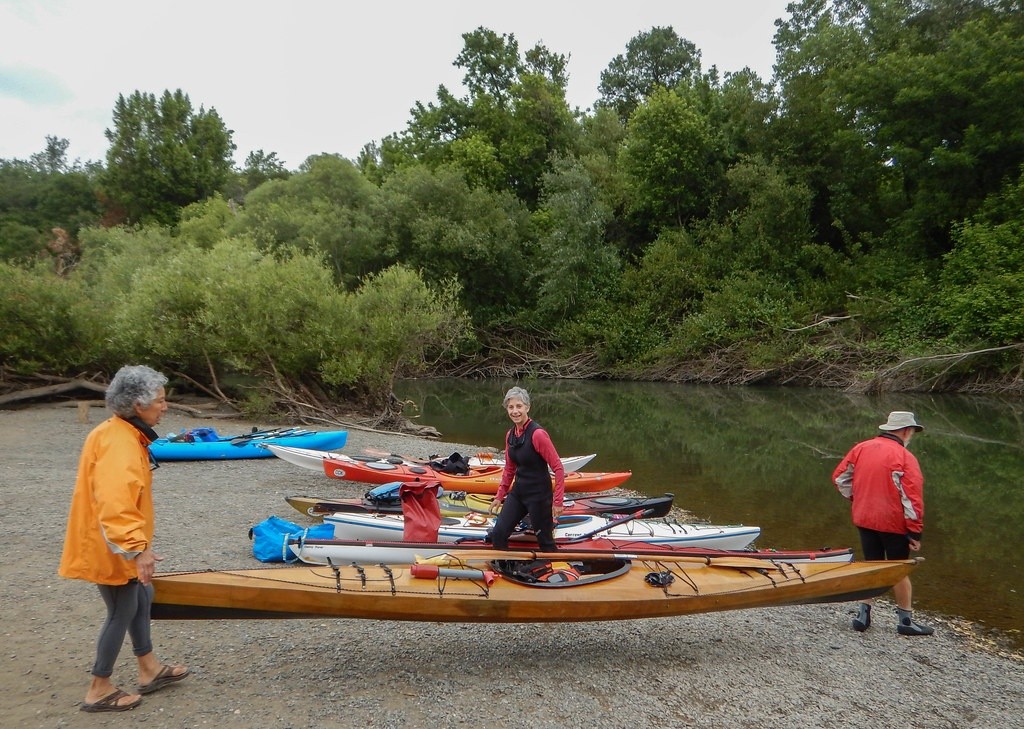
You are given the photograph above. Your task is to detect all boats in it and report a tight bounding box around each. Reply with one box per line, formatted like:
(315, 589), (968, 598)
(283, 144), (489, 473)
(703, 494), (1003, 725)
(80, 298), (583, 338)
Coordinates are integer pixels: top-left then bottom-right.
(288, 536), (854, 574)
(258, 441), (598, 472)
(152, 548), (925, 624)
(285, 492), (674, 519)
(322, 514), (760, 549)
(147, 428), (349, 461)
(322, 452), (633, 496)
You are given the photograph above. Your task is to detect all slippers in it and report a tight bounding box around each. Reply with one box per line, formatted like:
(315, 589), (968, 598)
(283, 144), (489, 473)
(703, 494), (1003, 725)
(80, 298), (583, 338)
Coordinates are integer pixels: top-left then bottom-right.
(136, 664), (190, 695)
(78, 687), (143, 712)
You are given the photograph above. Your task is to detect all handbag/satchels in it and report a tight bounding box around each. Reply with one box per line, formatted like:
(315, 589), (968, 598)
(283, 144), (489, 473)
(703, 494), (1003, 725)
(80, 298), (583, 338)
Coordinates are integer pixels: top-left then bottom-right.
(247, 515), (336, 564)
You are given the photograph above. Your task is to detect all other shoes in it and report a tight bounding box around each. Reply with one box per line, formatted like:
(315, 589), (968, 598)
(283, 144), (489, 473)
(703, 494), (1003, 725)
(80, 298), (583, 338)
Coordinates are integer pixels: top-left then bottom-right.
(897, 621), (934, 635)
(852, 610), (871, 631)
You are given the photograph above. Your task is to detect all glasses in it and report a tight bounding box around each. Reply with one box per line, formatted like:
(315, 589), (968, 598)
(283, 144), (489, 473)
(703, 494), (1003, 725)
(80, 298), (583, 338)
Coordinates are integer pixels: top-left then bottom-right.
(507, 433), (526, 448)
(139, 441), (160, 471)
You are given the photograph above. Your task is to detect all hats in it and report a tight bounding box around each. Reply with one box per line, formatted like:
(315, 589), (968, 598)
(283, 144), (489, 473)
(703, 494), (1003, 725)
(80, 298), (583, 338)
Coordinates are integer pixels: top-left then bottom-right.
(878, 411), (924, 432)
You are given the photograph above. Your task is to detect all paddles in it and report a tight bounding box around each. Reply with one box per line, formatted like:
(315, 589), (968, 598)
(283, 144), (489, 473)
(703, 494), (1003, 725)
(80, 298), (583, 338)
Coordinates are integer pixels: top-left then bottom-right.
(563, 494), (612, 502)
(571, 509), (654, 541)
(313, 502), (467, 516)
(446, 548), (777, 571)
(218, 427), (306, 445)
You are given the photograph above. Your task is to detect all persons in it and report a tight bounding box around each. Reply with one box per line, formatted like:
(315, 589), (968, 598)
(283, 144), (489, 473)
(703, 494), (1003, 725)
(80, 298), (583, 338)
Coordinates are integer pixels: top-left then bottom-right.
(832, 411), (935, 635)
(489, 386), (564, 568)
(56, 362), (193, 714)
